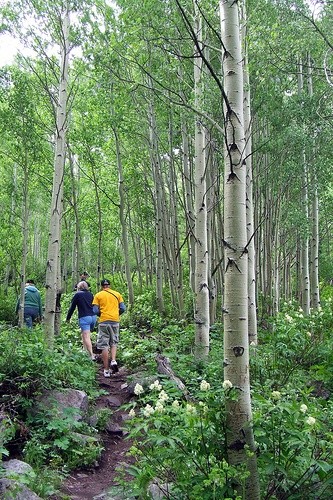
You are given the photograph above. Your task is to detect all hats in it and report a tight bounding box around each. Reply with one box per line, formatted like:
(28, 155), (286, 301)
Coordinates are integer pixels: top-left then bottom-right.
(26, 280), (34, 284)
(101, 279), (110, 285)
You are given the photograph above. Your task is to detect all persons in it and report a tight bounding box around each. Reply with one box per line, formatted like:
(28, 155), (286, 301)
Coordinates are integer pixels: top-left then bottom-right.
(15, 279), (43, 329)
(65, 281), (97, 360)
(92, 278), (125, 378)
(74, 275), (91, 293)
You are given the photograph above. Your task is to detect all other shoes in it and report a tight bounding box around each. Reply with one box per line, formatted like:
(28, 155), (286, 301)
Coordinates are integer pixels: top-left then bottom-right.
(91, 356), (95, 360)
(110, 361), (117, 367)
(103, 369), (111, 377)
